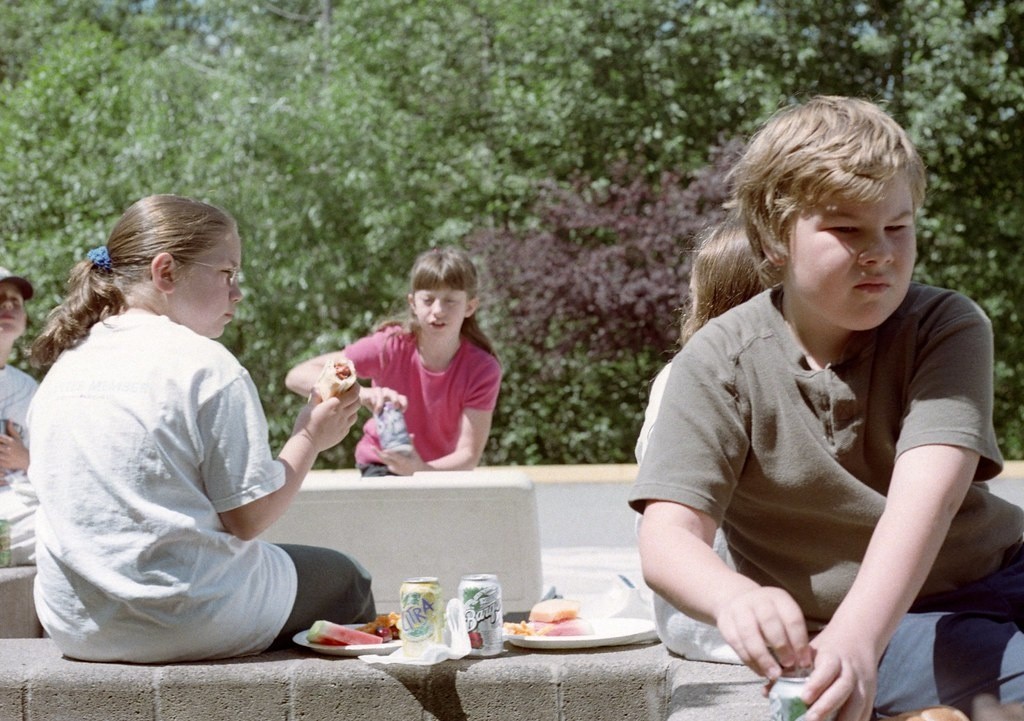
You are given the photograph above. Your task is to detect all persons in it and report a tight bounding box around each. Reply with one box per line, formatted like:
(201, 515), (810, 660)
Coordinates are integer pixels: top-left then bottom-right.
(27, 193), (377, 663)
(0, 266), (39, 567)
(634, 212), (768, 666)
(286, 247), (501, 477)
(629, 95), (1024, 720)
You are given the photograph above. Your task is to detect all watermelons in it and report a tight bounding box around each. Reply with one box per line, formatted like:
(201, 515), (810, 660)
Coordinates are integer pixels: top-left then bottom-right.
(306, 619), (382, 647)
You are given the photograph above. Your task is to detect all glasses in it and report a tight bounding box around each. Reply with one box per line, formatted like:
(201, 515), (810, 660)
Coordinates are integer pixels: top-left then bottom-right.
(171, 254), (240, 286)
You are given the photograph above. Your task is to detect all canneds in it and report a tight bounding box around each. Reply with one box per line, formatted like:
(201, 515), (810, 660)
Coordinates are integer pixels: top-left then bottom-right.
(458, 574), (504, 659)
(767, 660), (837, 721)
(374, 401), (413, 456)
(400, 576), (444, 661)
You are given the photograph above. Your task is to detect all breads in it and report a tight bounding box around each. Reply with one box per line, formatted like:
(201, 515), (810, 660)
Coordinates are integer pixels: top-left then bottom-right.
(874, 704), (970, 721)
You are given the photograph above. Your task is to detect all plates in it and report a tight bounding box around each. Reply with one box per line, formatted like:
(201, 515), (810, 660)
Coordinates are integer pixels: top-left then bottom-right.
(292, 623), (405, 655)
(501, 618), (657, 648)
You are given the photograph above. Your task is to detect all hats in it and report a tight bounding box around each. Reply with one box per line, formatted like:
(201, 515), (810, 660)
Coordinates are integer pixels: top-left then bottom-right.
(0, 266), (34, 300)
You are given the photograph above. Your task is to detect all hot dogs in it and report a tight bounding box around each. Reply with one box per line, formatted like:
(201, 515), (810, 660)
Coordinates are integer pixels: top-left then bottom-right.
(307, 358), (357, 404)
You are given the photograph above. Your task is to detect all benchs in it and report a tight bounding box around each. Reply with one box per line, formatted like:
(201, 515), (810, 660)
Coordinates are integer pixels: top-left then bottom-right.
(0, 563), (822, 721)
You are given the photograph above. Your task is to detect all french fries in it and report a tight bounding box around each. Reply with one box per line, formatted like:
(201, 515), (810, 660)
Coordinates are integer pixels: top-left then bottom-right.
(502, 619), (533, 636)
